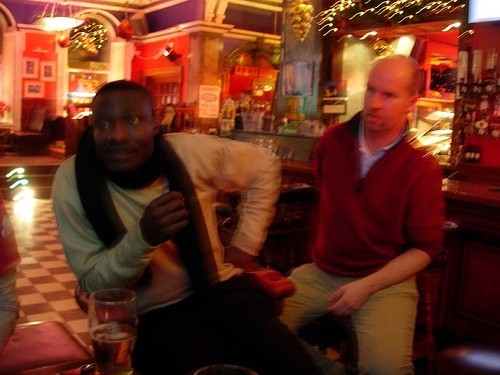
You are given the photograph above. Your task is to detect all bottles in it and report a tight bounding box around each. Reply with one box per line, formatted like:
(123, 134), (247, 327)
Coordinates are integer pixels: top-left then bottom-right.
(220, 97), (235, 131)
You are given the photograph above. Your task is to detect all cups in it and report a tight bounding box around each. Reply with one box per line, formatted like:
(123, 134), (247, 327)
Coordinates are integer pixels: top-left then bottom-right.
(89, 288), (138, 375)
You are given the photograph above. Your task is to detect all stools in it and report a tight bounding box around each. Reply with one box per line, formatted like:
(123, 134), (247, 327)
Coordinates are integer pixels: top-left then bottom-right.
(0, 261), (500, 375)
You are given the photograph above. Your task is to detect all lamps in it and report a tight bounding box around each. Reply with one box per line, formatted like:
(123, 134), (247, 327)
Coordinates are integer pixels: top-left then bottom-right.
(32, 0), (85, 33)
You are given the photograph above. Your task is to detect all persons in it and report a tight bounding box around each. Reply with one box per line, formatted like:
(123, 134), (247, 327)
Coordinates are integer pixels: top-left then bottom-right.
(279, 55), (445, 375)
(64, 99), (76, 116)
(52, 79), (325, 375)
(158, 103), (178, 133)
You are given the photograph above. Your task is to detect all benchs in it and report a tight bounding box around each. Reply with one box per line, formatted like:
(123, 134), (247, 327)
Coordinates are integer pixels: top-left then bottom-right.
(12, 107), (49, 155)
